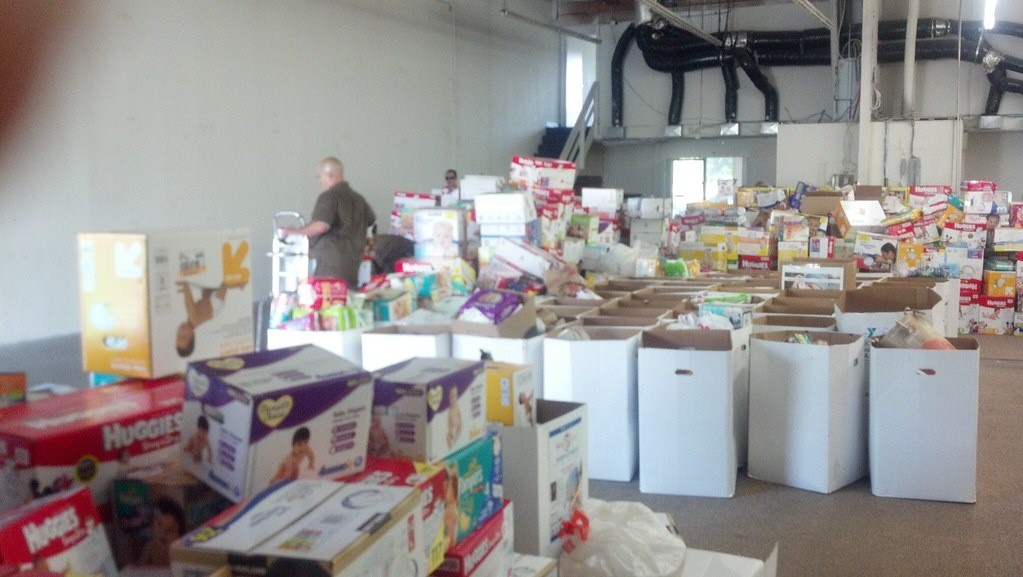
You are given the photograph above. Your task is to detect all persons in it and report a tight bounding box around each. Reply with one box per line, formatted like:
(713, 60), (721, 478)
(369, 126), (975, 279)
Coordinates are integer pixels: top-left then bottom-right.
(518, 391), (538, 426)
(368, 408), (390, 453)
(882, 243), (896, 262)
(443, 169), (458, 192)
(446, 385), (462, 448)
(270, 425), (316, 484)
(278, 157), (377, 291)
(986, 213), (1001, 229)
(808, 217), (822, 237)
(984, 307), (1000, 319)
(175, 282), (228, 356)
(183, 416), (213, 462)
(442, 472), (463, 543)
(372, 233), (414, 275)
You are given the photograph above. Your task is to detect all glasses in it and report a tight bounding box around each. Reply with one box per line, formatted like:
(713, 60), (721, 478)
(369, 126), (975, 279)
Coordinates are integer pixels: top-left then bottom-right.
(445, 176), (455, 179)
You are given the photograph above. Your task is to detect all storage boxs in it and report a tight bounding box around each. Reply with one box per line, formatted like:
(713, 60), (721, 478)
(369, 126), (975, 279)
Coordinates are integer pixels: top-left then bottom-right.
(0, 224), (781, 577)
(266, 155), (1023, 503)
